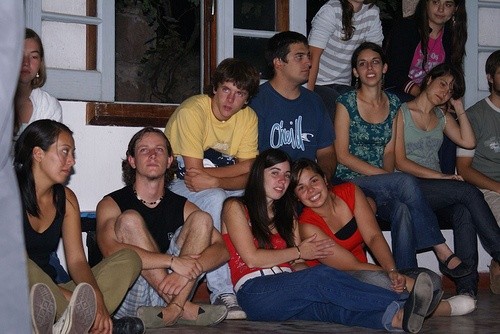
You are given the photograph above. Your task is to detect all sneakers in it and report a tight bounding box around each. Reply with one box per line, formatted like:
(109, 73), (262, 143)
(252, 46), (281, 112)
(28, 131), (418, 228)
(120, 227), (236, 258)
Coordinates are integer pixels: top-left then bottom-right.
(31, 281), (57, 334)
(51, 281), (98, 334)
(213, 291), (247, 320)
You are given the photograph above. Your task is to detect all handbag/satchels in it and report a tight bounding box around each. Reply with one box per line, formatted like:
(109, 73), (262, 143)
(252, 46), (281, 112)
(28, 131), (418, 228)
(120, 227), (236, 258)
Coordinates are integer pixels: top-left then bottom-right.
(177, 147), (240, 180)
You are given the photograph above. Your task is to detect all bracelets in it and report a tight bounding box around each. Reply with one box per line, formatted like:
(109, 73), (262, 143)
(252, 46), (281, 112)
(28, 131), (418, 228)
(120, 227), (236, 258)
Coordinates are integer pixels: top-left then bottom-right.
(295, 245), (301, 260)
(455, 110), (466, 120)
(169, 255), (175, 271)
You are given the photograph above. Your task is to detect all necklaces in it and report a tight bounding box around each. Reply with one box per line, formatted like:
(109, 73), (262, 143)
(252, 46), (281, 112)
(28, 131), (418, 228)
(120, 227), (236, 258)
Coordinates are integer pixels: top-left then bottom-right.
(133, 186), (168, 205)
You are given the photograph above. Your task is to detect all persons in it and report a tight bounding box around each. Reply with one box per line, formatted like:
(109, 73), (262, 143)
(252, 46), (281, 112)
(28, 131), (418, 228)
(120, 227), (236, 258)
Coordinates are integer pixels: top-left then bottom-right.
(297, 0), (500, 315)
(9, 27), (63, 155)
(243, 29), (338, 187)
(217, 149), (437, 333)
(149, 54), (259, 320)
(96, 126), (231, 326)
(7, 119), (142, 334)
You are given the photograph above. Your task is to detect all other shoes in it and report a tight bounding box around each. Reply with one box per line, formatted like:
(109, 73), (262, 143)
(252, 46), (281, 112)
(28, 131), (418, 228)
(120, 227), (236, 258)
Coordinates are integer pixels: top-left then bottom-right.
(461, 289), (476, 300)
(441, 294), (476, 317)
(489, 259), (500, 294)
(113, 315), (146, 334)
(439, 253), (473, 278)
(402, 271), (444, 334)
(137, 305), (178, 328)
(176, 303), (228, 326)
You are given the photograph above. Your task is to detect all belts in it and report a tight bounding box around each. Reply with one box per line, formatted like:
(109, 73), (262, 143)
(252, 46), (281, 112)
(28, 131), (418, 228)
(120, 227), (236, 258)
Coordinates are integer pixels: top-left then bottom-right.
(233, 264), (292, 292)
